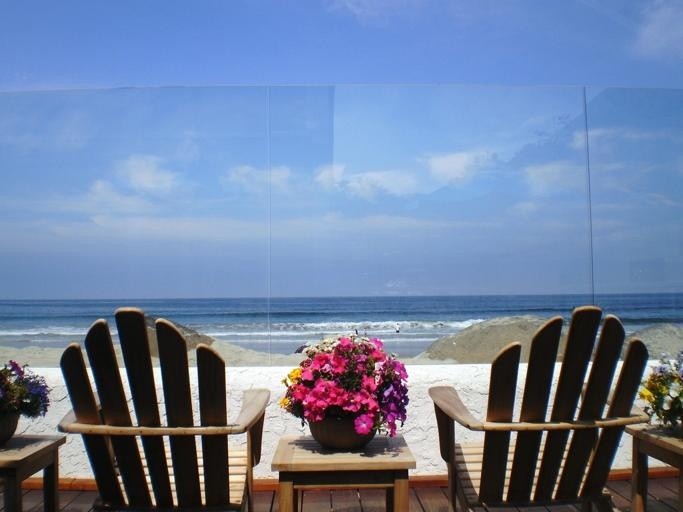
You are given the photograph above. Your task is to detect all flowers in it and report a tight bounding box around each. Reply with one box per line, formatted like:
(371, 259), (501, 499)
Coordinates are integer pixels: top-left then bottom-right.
(281, 334), (408, 436)
(0, 360), (49, 419)
(642, 355), (682, 435)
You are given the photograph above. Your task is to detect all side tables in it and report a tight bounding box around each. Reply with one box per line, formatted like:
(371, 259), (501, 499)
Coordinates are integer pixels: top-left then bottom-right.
(625, 426), (683, 511)
(1, 434), (66, 512)
(271, 436), (416, 512)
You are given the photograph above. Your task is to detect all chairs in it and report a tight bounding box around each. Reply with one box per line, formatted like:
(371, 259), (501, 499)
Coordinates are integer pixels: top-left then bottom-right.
(58, 308), (271, 512)
(429, 306), (649, 511)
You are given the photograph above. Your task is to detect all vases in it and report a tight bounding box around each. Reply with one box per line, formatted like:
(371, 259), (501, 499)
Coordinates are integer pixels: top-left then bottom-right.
(309, 414), (378, 450)
(0, 413), (20, 443)
